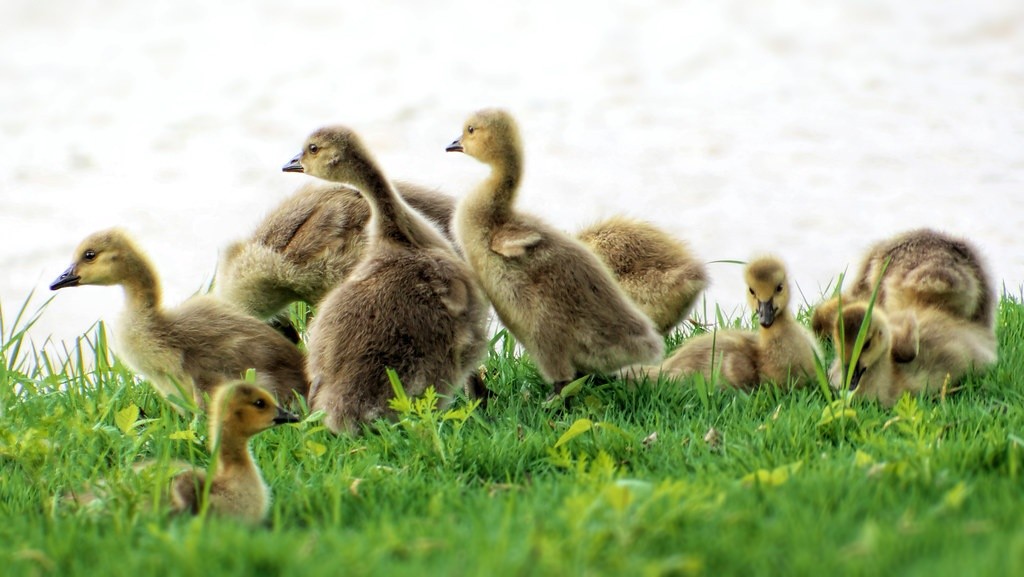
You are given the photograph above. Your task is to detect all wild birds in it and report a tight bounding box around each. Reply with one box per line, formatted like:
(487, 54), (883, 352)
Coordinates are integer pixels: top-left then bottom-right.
(574, 215), (709, 332)
(282, 125), (487, 438)
(830, 302), (996, 409)
(65, 380), (300, 526)
(662, 255), (823, 388)
(811, 227), (997, 388)
(445, 106), (663, 415)
(50, 228), (309, 411)
(216, 182), (460, 322)
